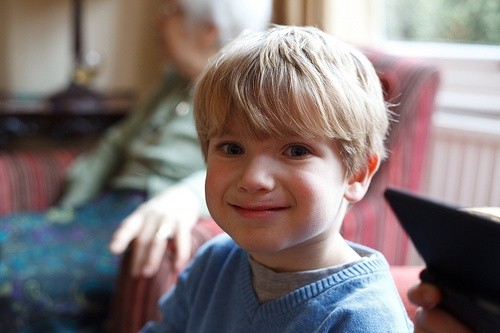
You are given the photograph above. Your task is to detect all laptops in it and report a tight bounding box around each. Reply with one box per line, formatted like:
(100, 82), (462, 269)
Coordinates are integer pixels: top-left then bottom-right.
(384, 186), (499, 333)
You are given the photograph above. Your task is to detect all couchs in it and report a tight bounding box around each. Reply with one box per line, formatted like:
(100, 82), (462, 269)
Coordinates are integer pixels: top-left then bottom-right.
(2, 37), (443, 331)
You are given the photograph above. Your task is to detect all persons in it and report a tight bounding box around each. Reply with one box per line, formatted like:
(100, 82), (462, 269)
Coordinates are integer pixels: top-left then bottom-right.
(1, 0), (274, 333)
(138, 21), (415, 333)
